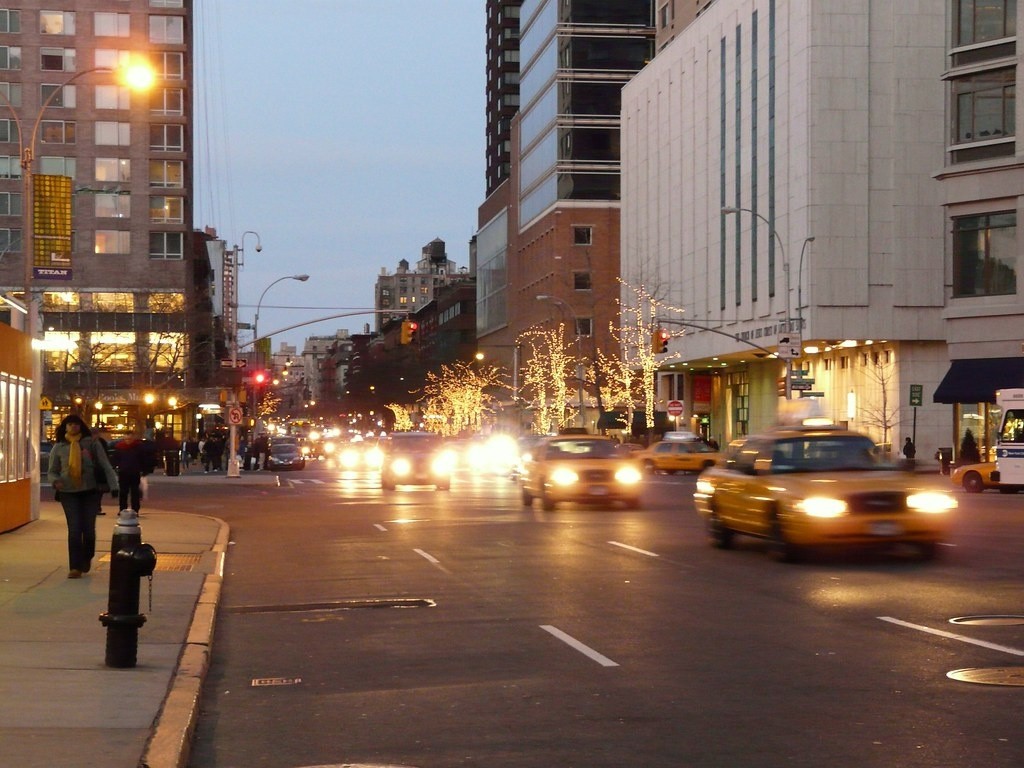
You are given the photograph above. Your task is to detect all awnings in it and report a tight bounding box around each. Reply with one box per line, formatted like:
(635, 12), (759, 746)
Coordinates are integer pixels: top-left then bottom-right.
(932, 357), (1024, 404)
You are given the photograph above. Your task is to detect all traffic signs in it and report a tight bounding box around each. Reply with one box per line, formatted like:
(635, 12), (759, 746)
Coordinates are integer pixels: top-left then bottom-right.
(221, 358), (248, 370)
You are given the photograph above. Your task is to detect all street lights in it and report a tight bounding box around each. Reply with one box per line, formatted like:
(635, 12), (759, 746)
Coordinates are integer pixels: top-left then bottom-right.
(76, 399), (82, 418)
(475, 345), (518, 404)
(96, 403), (102, 427)
(0, 57), (158, 522)
(253, 275), (310, 418)
(537, 296), (586, 427)
(228, 231), (263, 477)
(146, 395), (155, 437)
(722, 206), (794, 402)
(169, 398), (176, 439)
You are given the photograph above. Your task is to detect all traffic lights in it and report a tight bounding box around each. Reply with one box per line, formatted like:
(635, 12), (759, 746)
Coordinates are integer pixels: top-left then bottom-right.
(242, 416), (255, 429)
(251, 367), (269, 408)
(407, 323), (420, 346)
(651, 329), (669, 354)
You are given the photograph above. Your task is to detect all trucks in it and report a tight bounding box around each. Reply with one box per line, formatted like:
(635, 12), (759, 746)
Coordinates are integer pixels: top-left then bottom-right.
(996, 388), (1024, 493)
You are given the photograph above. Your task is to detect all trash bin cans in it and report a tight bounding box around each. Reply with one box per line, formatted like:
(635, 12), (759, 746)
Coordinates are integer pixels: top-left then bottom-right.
(938, 448), (953, 475)
(164, 449), (179, 476)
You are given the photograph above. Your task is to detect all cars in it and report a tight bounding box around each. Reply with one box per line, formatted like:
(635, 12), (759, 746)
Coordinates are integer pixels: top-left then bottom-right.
(107, 438), (157, 476)
(382, 435), (450, 493)
(266, 443), (306, 470)
(522, 430), (645, 510)
(694, 425), (958, 564)
(270, 419), (513, 488)
(954, 460), (997, 493)
(641, 432), (719, 475)
(40, 441), (54, 479)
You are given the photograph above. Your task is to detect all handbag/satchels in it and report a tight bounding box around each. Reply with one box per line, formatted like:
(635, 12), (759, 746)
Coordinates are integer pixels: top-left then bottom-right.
(90, 436), (111, 493)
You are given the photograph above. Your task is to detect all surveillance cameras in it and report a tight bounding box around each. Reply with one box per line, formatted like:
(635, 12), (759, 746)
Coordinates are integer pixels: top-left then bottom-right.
(256, 245), (262, 252)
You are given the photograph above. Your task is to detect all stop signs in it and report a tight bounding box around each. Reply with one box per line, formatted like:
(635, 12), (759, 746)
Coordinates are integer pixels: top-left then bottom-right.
(666, 400), (685, 421)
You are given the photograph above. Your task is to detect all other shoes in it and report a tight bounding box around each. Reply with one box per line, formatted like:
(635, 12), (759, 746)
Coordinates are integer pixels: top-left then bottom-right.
(81, 557), (90, 573)
(68, 568), (81, 578)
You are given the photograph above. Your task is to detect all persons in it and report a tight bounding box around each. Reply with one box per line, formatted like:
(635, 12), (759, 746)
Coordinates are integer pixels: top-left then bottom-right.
(903, 438), (916, 458)
(700, 433), (719, 450)
(164, 428), (269, 475)
(611, 434), (620, 445)
(47, 413), (119, 578)
(113, 430), (148, 518)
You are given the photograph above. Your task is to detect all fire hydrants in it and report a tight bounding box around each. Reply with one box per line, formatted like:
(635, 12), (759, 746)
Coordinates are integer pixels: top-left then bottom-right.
(98, 508), (156, 668)
